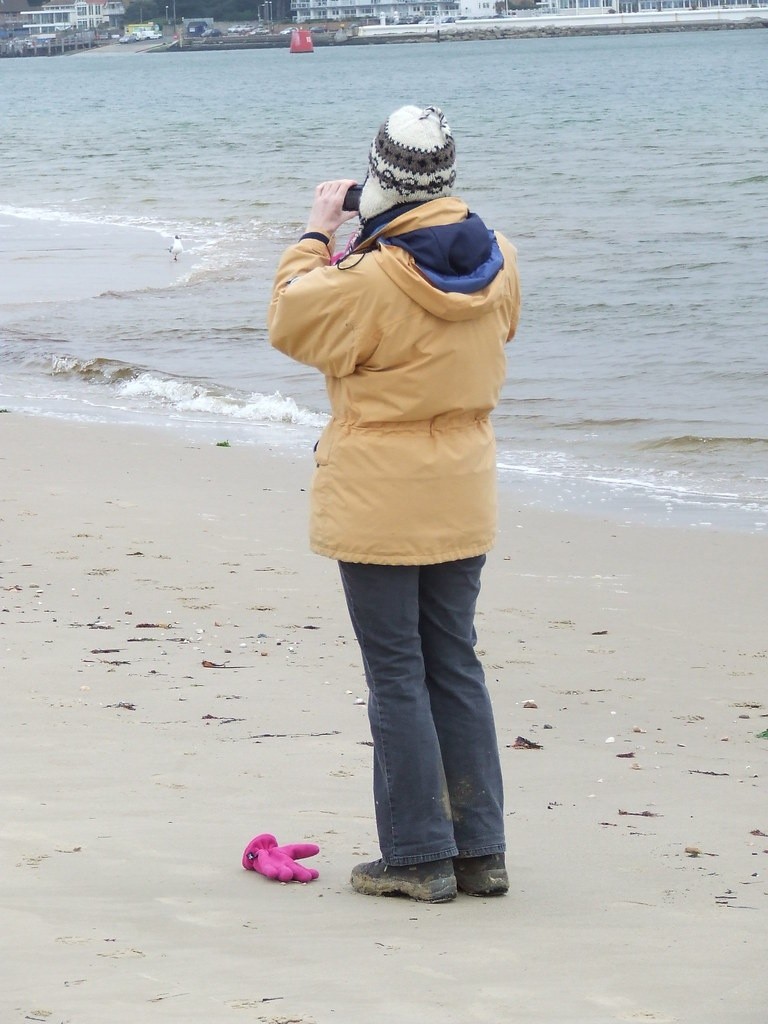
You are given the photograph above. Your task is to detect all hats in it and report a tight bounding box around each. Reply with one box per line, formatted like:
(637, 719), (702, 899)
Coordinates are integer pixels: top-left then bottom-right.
(360, 106), (454, 221)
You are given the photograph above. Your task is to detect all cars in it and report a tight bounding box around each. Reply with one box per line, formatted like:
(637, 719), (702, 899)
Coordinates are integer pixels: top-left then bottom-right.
(201, 29), (222, 37)
(279, 27), (297, 35)
(119, 31), (145, 43)
(311, 26), (327, 34)
(228, 24), (254, 33)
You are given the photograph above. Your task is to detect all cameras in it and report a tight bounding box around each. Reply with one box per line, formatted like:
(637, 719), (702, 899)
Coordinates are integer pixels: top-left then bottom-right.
(341, 185), (364, 212)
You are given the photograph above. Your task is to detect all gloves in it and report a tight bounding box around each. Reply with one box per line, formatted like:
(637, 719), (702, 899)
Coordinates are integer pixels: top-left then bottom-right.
(241, 832), (319, 883)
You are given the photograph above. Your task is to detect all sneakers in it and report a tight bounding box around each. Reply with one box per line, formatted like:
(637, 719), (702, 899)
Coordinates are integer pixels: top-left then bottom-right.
(349, 855), (458, 904)
(453, 848), (509, 896)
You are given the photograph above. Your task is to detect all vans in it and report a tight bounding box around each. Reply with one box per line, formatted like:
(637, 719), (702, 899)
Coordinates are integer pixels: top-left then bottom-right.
(143, 31), (162, 40)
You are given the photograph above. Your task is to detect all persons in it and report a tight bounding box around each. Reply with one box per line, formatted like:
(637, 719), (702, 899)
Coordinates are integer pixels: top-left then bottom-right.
(268, 104), (519, 903)
